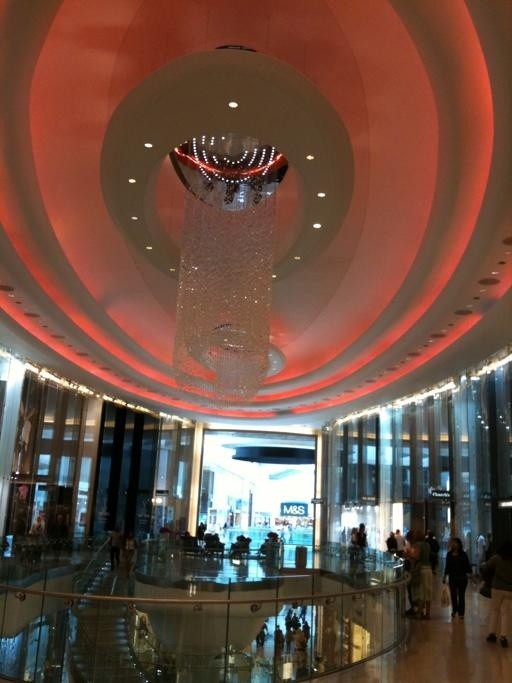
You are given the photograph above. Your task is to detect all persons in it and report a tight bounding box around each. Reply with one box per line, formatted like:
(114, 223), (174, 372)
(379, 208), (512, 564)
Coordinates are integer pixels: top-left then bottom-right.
(108, 527), (120, 570)
(348, 527), (358, 561)
(185, 531), (203, 548)
(256, 606), (310, 656)
(47, 513), (69, 550)
(197, 522), (206, 545)
(385, 528), (512, 648)
(122, 531), (138, 558)
(357, 523), (367, 558)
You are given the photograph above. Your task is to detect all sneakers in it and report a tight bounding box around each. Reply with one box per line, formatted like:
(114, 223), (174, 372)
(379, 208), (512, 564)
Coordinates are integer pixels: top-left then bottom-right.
(487, 635), (497, 643)
(406, 608), (430, 619)
(500, 639), (507, 648)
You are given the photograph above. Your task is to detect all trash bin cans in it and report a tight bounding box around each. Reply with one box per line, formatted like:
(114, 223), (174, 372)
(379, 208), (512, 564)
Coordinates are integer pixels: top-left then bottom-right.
(295, 546), (307, 568)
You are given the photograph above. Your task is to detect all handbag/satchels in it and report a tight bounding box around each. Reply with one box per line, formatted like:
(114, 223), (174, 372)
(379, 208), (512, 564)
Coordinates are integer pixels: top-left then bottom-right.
(480, 580), (491, 598)
(441, 584), (450, 608)
